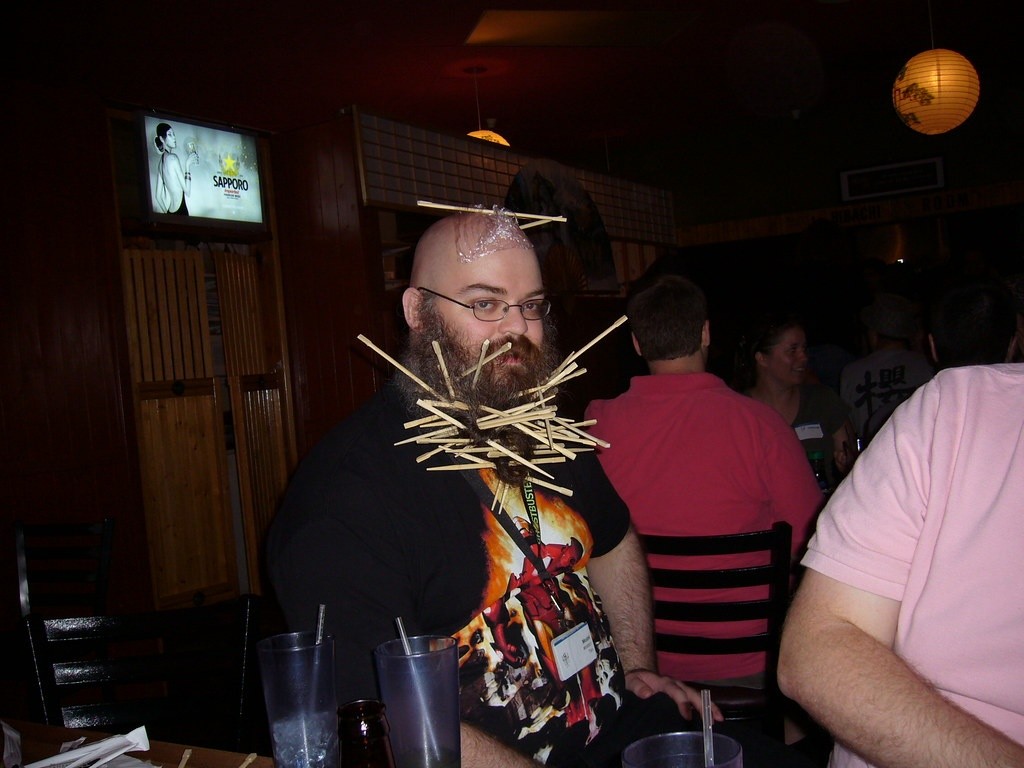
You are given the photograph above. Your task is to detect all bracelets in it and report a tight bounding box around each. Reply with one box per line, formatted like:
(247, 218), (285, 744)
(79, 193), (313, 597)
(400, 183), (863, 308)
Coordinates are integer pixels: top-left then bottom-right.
(625, 666), (653, 676)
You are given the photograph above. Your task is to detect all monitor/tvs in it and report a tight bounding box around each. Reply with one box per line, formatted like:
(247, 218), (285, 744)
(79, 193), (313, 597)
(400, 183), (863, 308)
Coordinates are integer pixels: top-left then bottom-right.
(138, 110), (273, 244)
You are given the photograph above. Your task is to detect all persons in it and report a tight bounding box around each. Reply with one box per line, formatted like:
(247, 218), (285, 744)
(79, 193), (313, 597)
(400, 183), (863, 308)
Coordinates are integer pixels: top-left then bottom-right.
(154, 123), (200, 216)
(775, 362), (1024, 768)
(577, 272), (1024, 747)
(259, 208), (724, 768)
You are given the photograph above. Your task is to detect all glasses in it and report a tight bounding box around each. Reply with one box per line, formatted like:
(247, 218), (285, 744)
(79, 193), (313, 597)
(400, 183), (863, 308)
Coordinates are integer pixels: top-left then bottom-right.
(417, 285), (552, 322)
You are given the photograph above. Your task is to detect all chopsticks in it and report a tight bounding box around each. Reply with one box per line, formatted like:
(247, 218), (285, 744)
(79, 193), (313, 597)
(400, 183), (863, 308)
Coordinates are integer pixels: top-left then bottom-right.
(177, 749), (257, 768)
(357, 201), (628, 516)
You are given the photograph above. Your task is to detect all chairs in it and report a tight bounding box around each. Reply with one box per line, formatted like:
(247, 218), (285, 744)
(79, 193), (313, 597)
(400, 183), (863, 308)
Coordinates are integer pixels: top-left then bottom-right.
(636, 521), (794, 768)
(0, 518), (256, 753)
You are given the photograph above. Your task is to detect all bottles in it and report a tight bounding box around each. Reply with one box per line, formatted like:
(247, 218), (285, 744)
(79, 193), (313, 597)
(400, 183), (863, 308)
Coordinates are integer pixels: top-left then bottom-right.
(853, 433), (864, 462)
(336, 698), (396, 768)
(808, 451), (824, 482)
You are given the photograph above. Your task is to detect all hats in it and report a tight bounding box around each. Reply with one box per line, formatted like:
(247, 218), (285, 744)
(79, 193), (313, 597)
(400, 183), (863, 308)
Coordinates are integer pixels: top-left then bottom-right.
(872, 293), (912, 337)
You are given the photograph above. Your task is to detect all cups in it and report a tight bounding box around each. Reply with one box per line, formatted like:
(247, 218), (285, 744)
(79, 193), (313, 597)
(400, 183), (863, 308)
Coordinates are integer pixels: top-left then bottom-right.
(622, 733), (743, 768)
(256, 631), (339, 768)
(376, 635), (461, 768)
